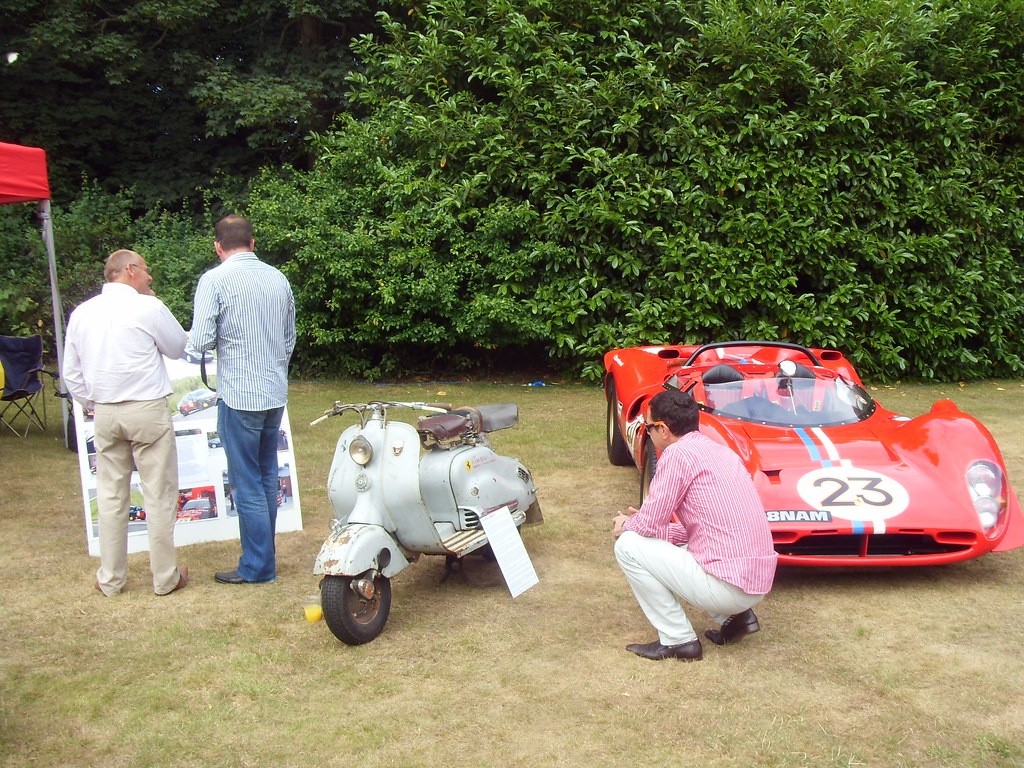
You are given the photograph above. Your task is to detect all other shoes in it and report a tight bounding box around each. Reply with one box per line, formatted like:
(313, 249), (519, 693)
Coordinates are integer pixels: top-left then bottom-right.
(95, 581), (122, 597)
(155, 565), (188, 596)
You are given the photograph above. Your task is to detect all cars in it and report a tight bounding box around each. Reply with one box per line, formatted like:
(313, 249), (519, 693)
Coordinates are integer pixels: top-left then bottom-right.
(208, 438), (223, 448)
(182, 498), (216, 519)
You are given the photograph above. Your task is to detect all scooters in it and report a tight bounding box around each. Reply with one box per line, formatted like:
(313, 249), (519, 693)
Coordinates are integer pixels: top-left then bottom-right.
(309, 399), (545, 648)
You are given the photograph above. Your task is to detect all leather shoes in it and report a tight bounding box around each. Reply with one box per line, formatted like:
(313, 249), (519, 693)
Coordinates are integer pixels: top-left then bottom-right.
(625, 639), (703, 663)
(215, 570), (276, 584)
(704, 608), (760, 646)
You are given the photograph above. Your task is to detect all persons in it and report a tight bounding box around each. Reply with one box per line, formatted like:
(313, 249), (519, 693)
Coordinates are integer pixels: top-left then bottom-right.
(612, 390), (780, 662)
(230, 493), (234, 510)
(191, 214), (297, 585)
(62, 249), (189, 598)
(282, 485), (288, 502)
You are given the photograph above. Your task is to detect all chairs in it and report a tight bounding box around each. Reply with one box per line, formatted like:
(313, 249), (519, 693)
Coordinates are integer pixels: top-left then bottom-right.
(0, 334), (48, 438)
(703, 361), (816, 411)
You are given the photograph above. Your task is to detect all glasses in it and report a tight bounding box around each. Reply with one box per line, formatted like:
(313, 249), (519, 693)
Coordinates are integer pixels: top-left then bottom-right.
(644, 420), (665, 435)
(126, 263), (151, 275)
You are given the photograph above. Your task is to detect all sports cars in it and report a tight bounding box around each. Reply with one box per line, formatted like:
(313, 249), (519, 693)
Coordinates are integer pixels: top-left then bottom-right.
(129, 506), (147, 522)
(177, 388), (216, 416)
(182, 343), (214, 364)
(602, 339), (1024, 573)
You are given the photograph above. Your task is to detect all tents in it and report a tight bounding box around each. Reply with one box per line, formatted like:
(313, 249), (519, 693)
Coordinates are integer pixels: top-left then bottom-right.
(1, 142), (71, 450)
